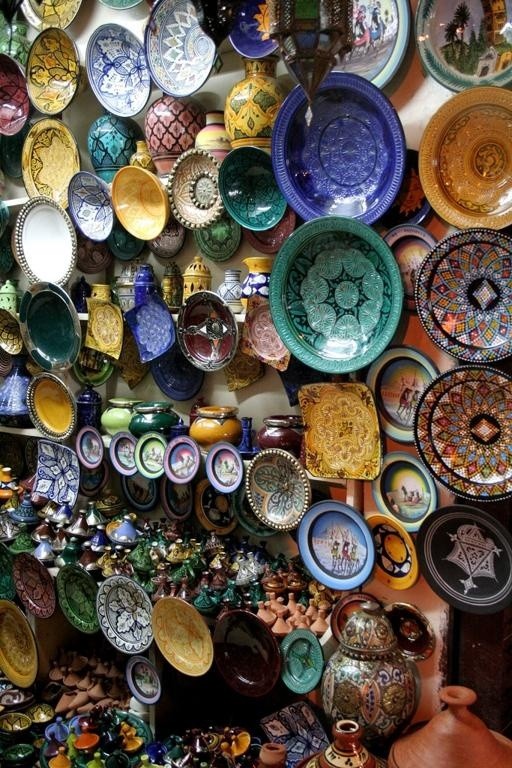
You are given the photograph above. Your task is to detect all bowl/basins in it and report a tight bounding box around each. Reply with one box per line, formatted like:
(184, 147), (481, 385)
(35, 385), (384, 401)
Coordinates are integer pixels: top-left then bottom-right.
(112, 166), (170, 241)
(68, 171), (116, 241)
(0, 742), (40, 767)
(0, 712), (32, 731)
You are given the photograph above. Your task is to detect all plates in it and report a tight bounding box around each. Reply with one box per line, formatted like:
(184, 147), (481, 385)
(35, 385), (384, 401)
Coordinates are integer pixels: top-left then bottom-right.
(379, 148), (431, 226)
(330, 592), (382, 642)
(193, 208), (243, 263)
(27, 372), (78, 442)
(85, 297), (124, 360)
(0, 676), (37, 705)
(415, 0), (512, 93)
(370, 450), (438, 532)
(241, 295), (291, 372)
(20, 0), (82, 32)
(0, 599), (40, 690)
(241, 205), (296, 254)
(15, 196), (77, 287)
(126, 655), (162, 704)
(72, 331), (114, 386)
(167, 149), (225, 231)
(218, 146), (287, 231)
(1, 307), (24, 355)
(76, 225), (114, 274)
(245, 448), (311, 532)
(0, 120), (33, 177)
(134, 431), (167, 479)
(195, 480), (237, 535)
(56, 564), (100, 634)
(1, 225), (15, 274)
(13, 551), (56, 619)
(20, 282), (81, 372)
(27, 27), (81, 117)
(26, 437), (39, 474)
(21, 118), (81, 210)
(96, 574), (154, 655)
(147, 211), (186, 259)
(418, 87), (511, 231)
(259, 701), (330, 768)
(297, 382), (382, 481)
(297, 500), (376, 591)
(414, 226), (512, 363)
(413, 365), (512, 503)
(0, 540), (16, 600)
(110, 431), (138, 476)
(151, 596), (213, 677)
(121, 471), (159, 512)
(104, 320), (151, 391)
(382, 223), (438, 312)
(0, 697), (38, 713)
(223, 322), (266, 392)
(76, 425), (104, 469)
(163, 436), (201, 485)
(268, 215), (403, 373)
(232, 483), (278, 536)
(144, 0), (218, 98)
(86, 23), (151, 118)
(108, 215), (145, 260)
(228, 0), (280, 59)
(159, 473), (195, 522)
(415, 504), (511, 616)
(176, 290), (239, 372)
(11, 228), (19, 263)
(123, 293), (176, 363)
(366, 346), (441, 444)
(205, 442), (243, 493)
(80, 457), (111, 497)
(364, 513), (419, 590)
(150, 340), (205, 401)
(97, 0), (143, 10)
(330, 0), (410, 90)
(381, 603), (436, 661)
(32, 439), (81, 509)
(213, 610), (281, 697)
(0, 52), (34, 136)
(272, 72), (405, 226)
(279, 628), (325, 695)
